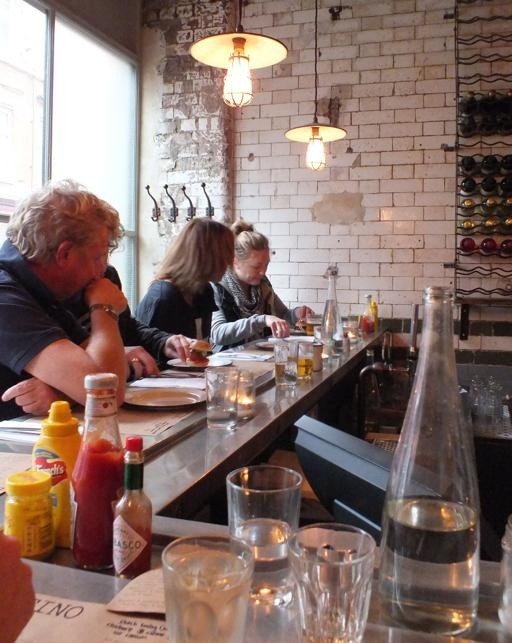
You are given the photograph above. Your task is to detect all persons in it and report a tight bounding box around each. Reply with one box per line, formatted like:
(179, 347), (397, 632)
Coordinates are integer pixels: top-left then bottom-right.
(212, 224), (316, 344)
(138, 220), (226, 353)
(90, 263), (210, 376)
(0, 181), (133, 415)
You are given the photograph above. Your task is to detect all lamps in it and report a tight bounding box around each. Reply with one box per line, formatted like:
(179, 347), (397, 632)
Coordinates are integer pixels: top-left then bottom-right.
(283, 0), (348, 170)
(189, 0), (288, 109)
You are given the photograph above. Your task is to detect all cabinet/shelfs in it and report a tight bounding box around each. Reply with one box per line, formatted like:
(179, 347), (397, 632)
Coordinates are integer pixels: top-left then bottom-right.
(472, 404), (512, 533)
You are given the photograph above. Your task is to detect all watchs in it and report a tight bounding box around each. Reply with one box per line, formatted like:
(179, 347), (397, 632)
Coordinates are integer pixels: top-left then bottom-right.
(87, 303), (125, 318)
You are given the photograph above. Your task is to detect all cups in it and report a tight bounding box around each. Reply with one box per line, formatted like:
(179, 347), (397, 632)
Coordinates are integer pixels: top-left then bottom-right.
(297, 340), (314, 380)
(225, 464), (303, 612)
(204, 366), (241, 431)
(285, 521), (377, 643)
(160, 533), (256, 642)
(306, 314), (322, 336)
(343, 321), (358, 344)
(238, 371), (257, 420)
(274, 342), (299, 387)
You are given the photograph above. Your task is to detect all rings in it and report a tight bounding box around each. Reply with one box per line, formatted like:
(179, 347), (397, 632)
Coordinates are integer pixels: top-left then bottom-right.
(128, 356), (146, 363)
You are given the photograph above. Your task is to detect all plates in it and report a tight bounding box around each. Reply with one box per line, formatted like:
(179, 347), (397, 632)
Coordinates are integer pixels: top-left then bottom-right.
(167, 357), (234, 370)
(121, 386), (206, 407)
(255, 341), (275, 349)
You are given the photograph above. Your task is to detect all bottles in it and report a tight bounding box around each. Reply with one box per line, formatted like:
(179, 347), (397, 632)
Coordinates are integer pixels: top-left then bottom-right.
(321, 270), (344, 358)
(367, 295), (372, 319)
(71, 374), (124, 571)
(4, 470), (55, 561)
(457, 88), (512, 256)
(31, 401), (82, 550)
(379, 284), (482, 636)
(111, 436), (152, 579)
(372, 301), (378, 333)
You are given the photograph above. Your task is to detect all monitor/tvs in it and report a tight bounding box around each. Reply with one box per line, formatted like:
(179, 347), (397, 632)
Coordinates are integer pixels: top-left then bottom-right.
(294, 416), (497, 560)
